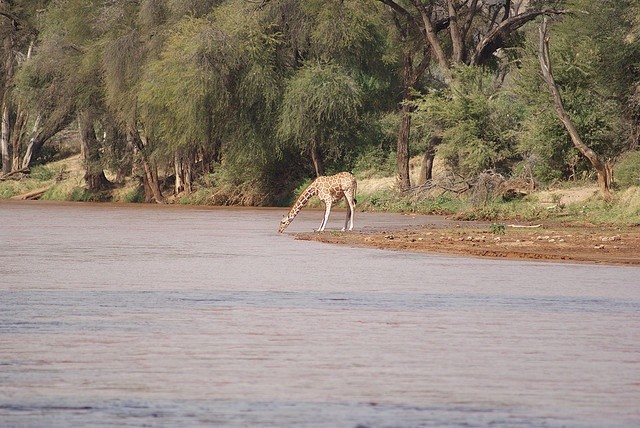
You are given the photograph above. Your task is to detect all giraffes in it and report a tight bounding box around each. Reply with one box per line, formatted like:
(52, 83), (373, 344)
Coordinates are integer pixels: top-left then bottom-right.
(278, 171), (357, 233)
